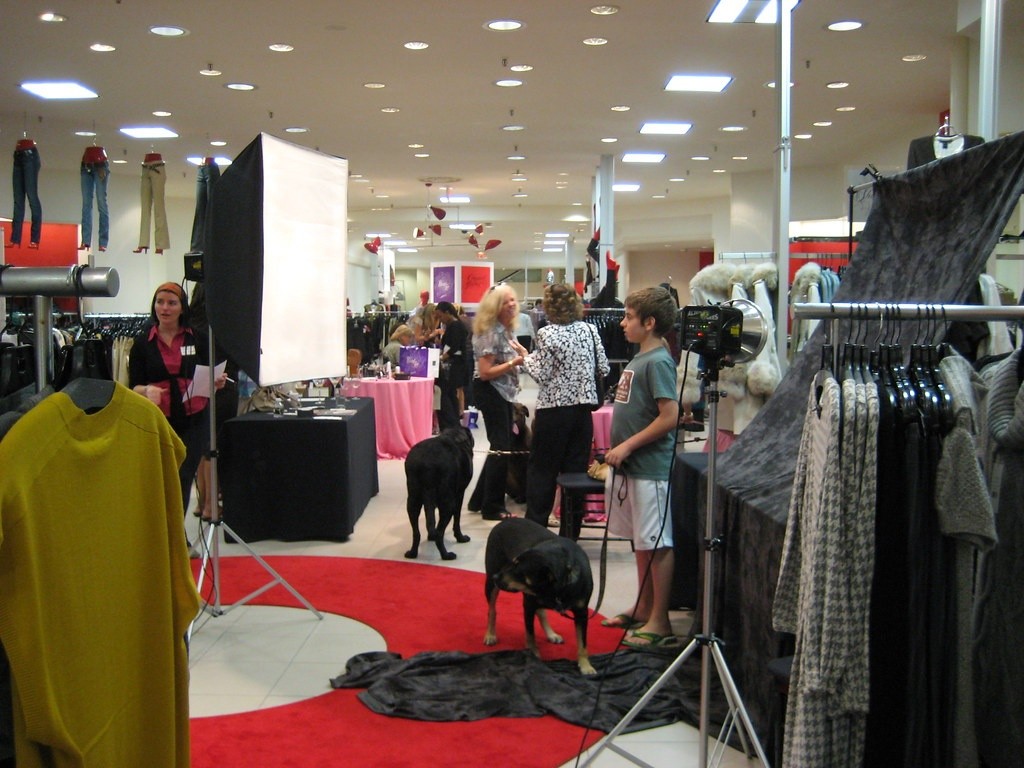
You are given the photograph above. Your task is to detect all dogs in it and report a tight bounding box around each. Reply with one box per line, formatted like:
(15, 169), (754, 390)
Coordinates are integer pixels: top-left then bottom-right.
(404, 425), (474, 560)
(505, 403), (536, 504)
(483, 518), (597, 675)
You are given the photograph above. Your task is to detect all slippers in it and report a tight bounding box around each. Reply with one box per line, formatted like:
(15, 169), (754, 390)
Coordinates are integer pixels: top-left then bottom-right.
(621, 629), (677, 649)
(602, 613), (647, 628)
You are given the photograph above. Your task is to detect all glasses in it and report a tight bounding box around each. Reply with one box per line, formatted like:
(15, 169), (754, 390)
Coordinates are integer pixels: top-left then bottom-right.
(489, 282), (502, 295)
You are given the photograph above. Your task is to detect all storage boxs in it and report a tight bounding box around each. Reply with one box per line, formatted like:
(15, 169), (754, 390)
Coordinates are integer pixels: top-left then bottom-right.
(399, 347), (440, 378)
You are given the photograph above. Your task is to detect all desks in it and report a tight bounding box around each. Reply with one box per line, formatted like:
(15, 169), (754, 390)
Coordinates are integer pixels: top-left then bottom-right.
(342, 377), (434, 460)
(555, 405), (614, 522)
(218, 395), (377, 545)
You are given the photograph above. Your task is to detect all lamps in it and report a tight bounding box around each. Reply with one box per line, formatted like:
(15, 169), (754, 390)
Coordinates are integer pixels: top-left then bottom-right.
(469, 235), (478, 248)
(485, 240), (501, 250)
(200, 64), (221, 76)
(427, 183), (446, 220)
(475, 224), (483, 235)
(428, 225), (441, 236)
(363, 234), (382, 254)
(413, 227), (425, 238)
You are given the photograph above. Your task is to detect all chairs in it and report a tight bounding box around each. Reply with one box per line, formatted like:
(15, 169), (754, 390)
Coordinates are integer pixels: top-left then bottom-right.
(558, 472), (634, 552)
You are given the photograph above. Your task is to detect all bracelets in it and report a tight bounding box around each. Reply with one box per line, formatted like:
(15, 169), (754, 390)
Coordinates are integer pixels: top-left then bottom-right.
(508, 361), (513, 366)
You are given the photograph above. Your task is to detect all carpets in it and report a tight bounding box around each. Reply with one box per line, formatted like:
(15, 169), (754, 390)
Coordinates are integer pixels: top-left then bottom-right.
(189, 556), (633, 768)
(806, 251), (848, 280)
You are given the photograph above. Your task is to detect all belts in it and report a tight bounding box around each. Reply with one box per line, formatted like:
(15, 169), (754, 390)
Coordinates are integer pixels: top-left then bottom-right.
(141, 163), (164, 173)
(14, 147), (36, 157)
(81, 161), (106, 168)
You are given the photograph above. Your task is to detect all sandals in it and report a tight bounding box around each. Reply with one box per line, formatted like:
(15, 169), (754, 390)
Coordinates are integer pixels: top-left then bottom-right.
(490, 511), (517, 520)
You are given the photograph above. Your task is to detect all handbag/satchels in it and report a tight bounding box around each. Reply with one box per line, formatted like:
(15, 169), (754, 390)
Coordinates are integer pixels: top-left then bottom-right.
(239, 386), (301, 416)
(462, 406), (479, 429)
(587, 323), (603, 411)
(399, 344), (439, 378)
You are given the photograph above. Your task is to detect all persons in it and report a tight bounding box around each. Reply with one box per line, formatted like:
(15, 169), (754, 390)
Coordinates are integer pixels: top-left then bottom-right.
(504, 282), (612, 543)
(78, 147), (111, 252)
(604, 285), (681, 648)
(4, 139), (43, 250)
(529, 299), (542, 334)
(133, 154), (170, 255)
(190, 157), (221, 253)
(194, 455), (222, 521)
(129, 282), (200, 558)
(381, 299), (473, 434)
(469, 281), (531, 521)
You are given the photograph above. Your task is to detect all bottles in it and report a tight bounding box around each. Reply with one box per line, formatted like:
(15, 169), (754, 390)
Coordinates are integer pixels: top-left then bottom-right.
(363, 364), (368, 377)
(386, 359), (392, 379)
(395, 364), (401, 374)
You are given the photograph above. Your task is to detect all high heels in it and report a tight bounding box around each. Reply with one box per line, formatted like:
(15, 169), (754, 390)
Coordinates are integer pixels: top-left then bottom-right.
(77, 243), (89, 251)
(5, 242), (20, 248)
(154, 248), (163, 254)
(133, 247), (147, 254)
(99, 246), (106, 252)
(27, 242), (39, 249)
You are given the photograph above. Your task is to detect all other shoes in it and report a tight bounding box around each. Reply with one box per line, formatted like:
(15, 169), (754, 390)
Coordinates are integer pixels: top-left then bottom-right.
(188, 547), (200, 558)
(193, 510), (202, 516)
(202, 515), (211, 521)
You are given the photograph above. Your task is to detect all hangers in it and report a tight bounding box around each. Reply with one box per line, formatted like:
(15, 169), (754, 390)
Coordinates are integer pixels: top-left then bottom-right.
(743, 251), (747, 263)
(718, 252), (725, 261)
(810, 302), (952, 416)
(347, 311), (411, 321)
(583, 309), (625, 328)
(0, 263), (149, 411)
(759, 251), (768, 263)
(935, 116), (959, 138)
